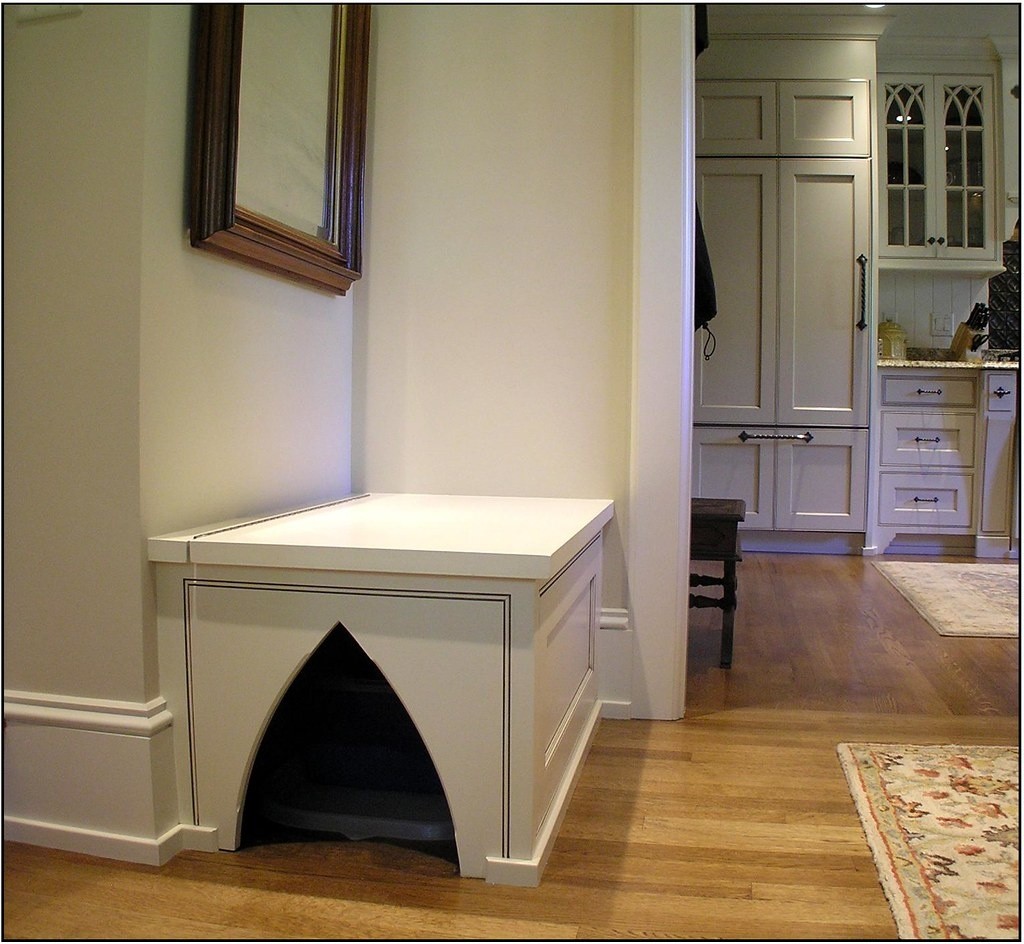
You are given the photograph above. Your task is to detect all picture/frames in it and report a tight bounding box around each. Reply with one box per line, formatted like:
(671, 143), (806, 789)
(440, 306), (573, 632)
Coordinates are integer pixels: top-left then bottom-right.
(189, 2), (372, 296)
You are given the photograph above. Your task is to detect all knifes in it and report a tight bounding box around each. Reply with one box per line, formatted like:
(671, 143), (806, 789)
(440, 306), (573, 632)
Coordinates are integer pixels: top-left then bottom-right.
(965, 302), (991, 332)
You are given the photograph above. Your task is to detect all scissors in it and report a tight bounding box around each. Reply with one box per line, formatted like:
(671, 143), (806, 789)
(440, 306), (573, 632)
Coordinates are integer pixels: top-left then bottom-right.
(971, 333), (989, 352)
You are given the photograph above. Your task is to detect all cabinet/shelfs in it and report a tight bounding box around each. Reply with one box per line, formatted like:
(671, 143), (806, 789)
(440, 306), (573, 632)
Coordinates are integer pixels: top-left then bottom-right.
(975, 370), (1020, 559)
(877, 365), (982, 557)
(876, 58), (1006, 281)
(692, 5), (897, 555)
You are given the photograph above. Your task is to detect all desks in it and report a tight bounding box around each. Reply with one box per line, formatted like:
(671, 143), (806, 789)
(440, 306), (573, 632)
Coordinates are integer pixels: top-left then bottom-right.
(148, 494), (613, 886)
(688, 497), (746, 668)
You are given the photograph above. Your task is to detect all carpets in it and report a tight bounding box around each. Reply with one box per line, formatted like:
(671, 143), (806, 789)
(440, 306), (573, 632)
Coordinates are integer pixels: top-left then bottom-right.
(871, 560), (1019, 638)
(836, 742), (1019, 940)
